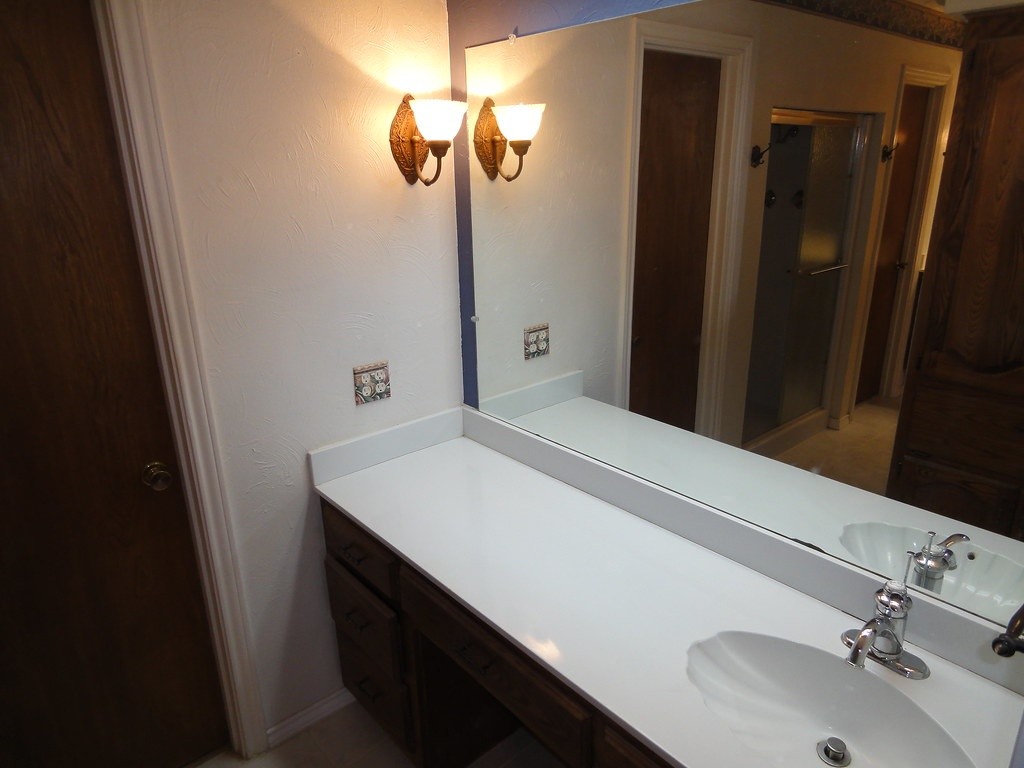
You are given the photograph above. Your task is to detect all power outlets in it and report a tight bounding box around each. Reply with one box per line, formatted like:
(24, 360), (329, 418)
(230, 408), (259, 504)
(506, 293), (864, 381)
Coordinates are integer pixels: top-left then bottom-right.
(522, 324), (552, 361)
(354, 361), (391, 404)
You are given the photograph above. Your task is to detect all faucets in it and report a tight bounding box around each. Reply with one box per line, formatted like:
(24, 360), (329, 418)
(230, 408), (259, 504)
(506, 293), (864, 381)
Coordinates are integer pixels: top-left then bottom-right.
(839, 578), (932, 680)
(911, 533), (970, 594)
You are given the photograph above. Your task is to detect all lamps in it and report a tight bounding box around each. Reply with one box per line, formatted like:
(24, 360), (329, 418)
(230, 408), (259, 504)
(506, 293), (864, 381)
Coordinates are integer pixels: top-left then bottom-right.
(389, 92), (468, 186)
(472, 93), (548, 187)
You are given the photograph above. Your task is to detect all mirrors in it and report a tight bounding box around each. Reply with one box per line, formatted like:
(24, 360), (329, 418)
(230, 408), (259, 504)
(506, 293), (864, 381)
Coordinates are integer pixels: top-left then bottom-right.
(459, 0), (1024, 627)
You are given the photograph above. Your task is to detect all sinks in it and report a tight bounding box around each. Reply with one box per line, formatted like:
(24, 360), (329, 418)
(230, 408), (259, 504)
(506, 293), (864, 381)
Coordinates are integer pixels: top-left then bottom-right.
(838, 520), (1024, 613)
(684, 628), (976, 768)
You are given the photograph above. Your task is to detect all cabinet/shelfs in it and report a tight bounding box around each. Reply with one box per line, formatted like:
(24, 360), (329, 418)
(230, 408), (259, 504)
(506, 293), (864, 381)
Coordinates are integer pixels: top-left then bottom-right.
(320, 503), (665, 768)
